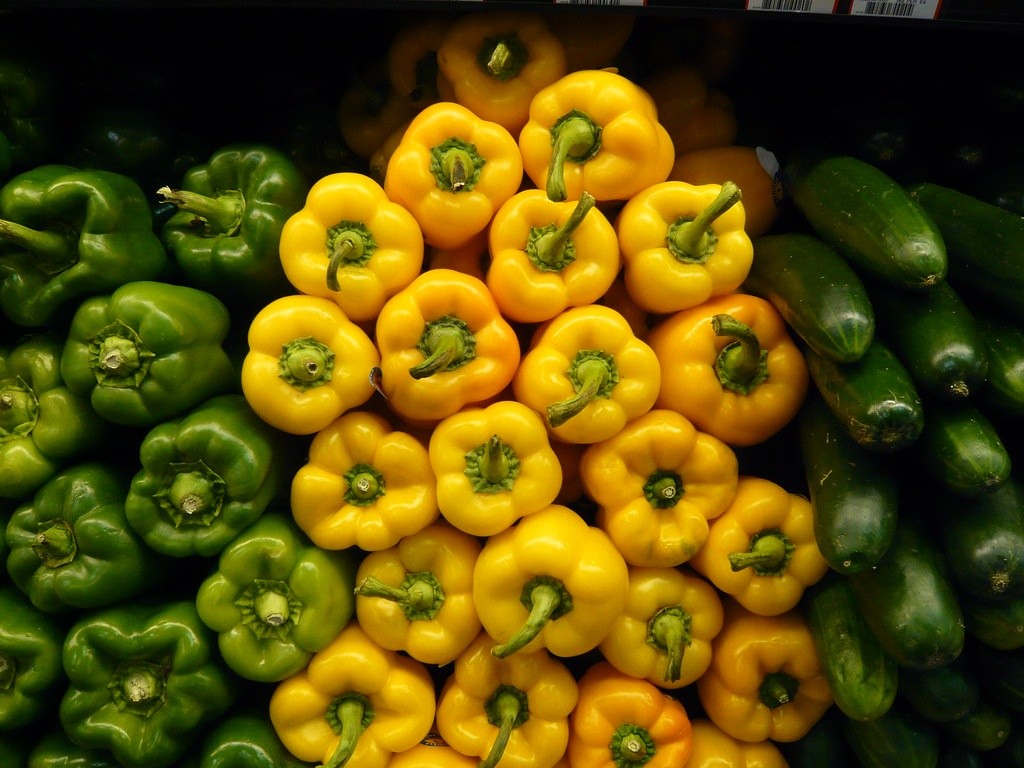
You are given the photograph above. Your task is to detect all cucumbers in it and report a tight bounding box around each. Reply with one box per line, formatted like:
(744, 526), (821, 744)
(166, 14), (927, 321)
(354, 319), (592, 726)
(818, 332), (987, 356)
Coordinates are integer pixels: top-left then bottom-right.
(749, 149), (1024, 768)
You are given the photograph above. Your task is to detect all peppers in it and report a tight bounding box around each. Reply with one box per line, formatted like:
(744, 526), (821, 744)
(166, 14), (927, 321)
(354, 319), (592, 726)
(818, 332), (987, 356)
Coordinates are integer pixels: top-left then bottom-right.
(0, 0), (832, 768)
